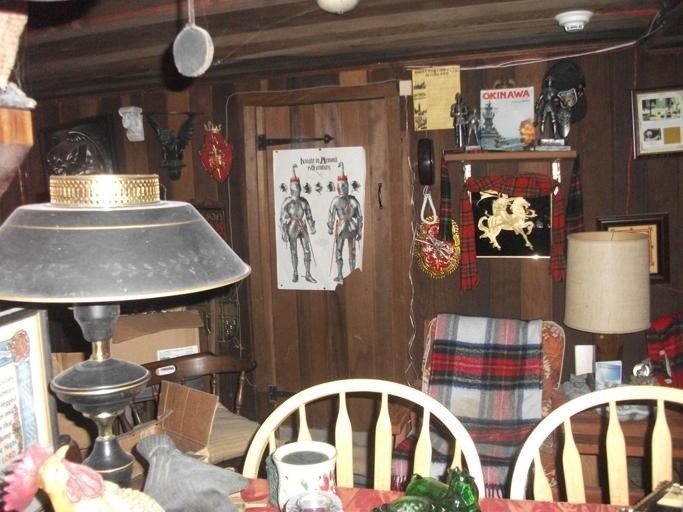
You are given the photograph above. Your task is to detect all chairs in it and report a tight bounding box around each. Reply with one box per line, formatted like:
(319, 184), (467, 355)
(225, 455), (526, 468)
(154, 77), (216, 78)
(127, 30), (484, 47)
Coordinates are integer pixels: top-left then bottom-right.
(130, 352), (257, 465)
(242, 378), (485, 497)
(510, 386), (683, 505)
(391, 313), (566, 498)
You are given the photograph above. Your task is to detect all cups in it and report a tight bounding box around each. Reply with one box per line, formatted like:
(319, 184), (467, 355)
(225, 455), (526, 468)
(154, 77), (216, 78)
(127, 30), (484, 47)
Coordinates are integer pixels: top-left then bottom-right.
(272, 439), (337, 512)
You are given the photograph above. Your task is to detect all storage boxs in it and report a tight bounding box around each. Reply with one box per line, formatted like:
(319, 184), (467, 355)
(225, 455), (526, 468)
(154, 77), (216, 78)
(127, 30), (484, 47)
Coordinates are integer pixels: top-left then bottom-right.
(117, 381), (219, 491)
(51, 353), (93, 460)
(111, 310), (203, 403)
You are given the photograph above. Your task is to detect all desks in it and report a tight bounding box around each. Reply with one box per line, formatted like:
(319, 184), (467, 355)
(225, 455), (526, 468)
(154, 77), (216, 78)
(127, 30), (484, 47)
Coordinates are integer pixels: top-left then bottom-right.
(557, 404), (683, 504)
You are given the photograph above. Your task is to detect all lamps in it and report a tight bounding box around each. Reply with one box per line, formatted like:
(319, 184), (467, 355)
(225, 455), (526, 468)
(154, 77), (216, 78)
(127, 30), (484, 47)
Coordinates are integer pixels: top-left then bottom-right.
(0, 175), (251, 488)
(563, 231), (651, 390)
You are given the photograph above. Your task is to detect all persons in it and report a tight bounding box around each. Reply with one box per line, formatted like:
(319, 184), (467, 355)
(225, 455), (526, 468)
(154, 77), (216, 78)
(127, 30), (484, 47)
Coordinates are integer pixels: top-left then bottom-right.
(468, 108), (480, 144)
(535, 74), (569, 141)
(451, 92), (469, 146)
(326, 161), (363, 283)
(280, 164), (317, 283)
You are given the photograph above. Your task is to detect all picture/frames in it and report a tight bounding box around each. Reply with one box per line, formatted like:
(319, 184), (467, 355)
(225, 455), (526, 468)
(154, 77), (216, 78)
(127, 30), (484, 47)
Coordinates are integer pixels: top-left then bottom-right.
(36, 112), (119, 192)
(596, 212), (670, 284)
(0, 308), (60, 512)
(631, 85), (683, 160)
